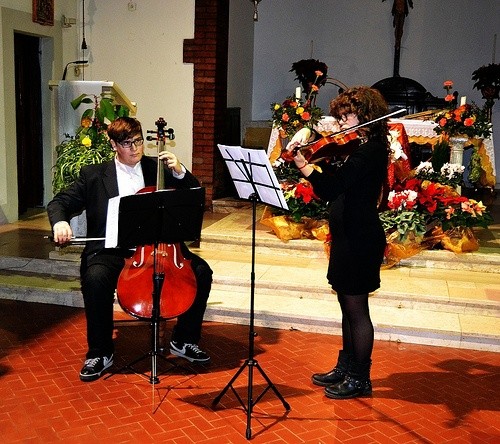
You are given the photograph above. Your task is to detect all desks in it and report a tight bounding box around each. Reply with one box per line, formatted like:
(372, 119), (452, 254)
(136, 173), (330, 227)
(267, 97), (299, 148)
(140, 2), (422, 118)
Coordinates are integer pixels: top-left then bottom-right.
(266, 115), (496, 197)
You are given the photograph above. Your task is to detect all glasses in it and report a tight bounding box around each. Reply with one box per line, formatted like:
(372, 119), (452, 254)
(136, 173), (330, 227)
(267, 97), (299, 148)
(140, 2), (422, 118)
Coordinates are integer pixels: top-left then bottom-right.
(116, 137), (144, 148)
(337, 111), (352, 123)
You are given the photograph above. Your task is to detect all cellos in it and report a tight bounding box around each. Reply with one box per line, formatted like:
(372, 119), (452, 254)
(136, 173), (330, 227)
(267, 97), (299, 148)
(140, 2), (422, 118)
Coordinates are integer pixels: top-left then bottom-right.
(116, 117), (197, 414)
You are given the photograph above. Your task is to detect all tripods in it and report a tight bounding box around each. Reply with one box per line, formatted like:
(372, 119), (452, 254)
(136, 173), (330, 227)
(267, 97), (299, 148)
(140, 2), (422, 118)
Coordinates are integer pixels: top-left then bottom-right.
(104, 186), (198, 415)
(217, 143), (293, 440)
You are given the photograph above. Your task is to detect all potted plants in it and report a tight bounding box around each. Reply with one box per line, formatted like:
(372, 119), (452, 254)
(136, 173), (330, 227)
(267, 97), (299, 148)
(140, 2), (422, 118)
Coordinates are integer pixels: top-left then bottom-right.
(472, 63), (500, 98)
(289, 58), (328, 92)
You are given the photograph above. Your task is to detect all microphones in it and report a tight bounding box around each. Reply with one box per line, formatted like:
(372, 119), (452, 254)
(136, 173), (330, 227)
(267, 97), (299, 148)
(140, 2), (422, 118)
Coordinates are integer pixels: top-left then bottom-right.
(62, 61), (88, 80)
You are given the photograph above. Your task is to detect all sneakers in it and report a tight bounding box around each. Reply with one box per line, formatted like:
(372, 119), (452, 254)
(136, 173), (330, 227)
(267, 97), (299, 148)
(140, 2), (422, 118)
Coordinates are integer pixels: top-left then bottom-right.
(170, 341), (210, 364)
(79, 354), (114, 380)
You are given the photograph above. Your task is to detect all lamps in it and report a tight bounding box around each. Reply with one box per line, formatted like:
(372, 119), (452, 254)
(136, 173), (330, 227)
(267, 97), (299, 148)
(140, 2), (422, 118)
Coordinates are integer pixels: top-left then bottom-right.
(61, 14), (76, 28)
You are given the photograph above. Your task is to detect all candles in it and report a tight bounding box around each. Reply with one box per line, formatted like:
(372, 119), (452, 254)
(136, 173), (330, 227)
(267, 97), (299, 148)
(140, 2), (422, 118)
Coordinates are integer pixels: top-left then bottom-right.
(492, 33), (497, 64)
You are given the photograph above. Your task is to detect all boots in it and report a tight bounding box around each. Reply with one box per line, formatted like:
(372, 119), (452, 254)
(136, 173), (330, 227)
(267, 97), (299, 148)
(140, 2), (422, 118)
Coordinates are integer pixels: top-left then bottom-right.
(325, 359), (372, 399)
(312, 350), (351, 386)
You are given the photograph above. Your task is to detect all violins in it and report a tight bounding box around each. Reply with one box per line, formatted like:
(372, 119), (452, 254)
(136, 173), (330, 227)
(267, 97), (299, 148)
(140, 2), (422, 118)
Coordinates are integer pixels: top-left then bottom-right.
(281, 129), (368, 169)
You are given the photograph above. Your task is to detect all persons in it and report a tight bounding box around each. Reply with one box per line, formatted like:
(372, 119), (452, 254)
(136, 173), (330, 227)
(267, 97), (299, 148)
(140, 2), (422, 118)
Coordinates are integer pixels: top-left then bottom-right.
(47, 117), (213, 381)
(286, 86), (392, 399)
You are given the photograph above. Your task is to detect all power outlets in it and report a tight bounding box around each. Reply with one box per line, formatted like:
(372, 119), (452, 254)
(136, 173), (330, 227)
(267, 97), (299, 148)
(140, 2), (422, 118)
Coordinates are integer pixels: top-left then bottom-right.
(128, 3), (136, 10)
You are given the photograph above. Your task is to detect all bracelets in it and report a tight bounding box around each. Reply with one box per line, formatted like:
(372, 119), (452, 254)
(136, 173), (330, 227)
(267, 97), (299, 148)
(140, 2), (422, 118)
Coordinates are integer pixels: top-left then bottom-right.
(298, 161), (308, 169)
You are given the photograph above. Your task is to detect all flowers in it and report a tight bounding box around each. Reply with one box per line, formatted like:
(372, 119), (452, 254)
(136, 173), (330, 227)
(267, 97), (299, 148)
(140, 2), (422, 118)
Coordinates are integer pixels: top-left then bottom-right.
(271, 71), (492, 245)
